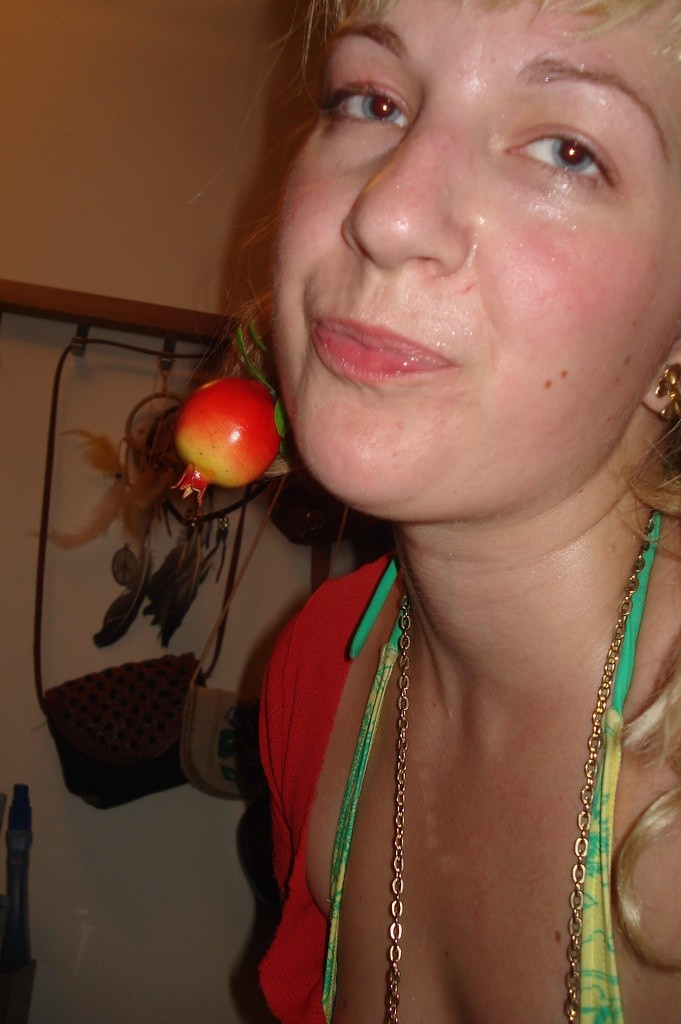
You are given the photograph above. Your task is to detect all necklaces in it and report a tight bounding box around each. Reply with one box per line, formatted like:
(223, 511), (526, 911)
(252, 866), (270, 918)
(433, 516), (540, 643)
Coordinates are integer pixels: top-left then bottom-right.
(383, 520), (655, 1024)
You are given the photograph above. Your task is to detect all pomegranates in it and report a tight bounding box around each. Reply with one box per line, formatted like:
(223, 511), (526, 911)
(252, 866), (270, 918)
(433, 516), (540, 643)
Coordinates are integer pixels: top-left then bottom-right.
(167, 376), (281, 503)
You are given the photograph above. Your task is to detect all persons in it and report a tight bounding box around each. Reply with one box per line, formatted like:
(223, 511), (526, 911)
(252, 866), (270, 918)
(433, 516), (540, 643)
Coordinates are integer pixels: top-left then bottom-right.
(141, 0), (681, 1024)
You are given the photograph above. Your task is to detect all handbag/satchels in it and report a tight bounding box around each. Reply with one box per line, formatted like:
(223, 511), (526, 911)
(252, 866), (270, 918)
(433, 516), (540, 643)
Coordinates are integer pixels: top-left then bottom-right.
(40, 652), (206, 808)
(181, 683), (271, 799)
